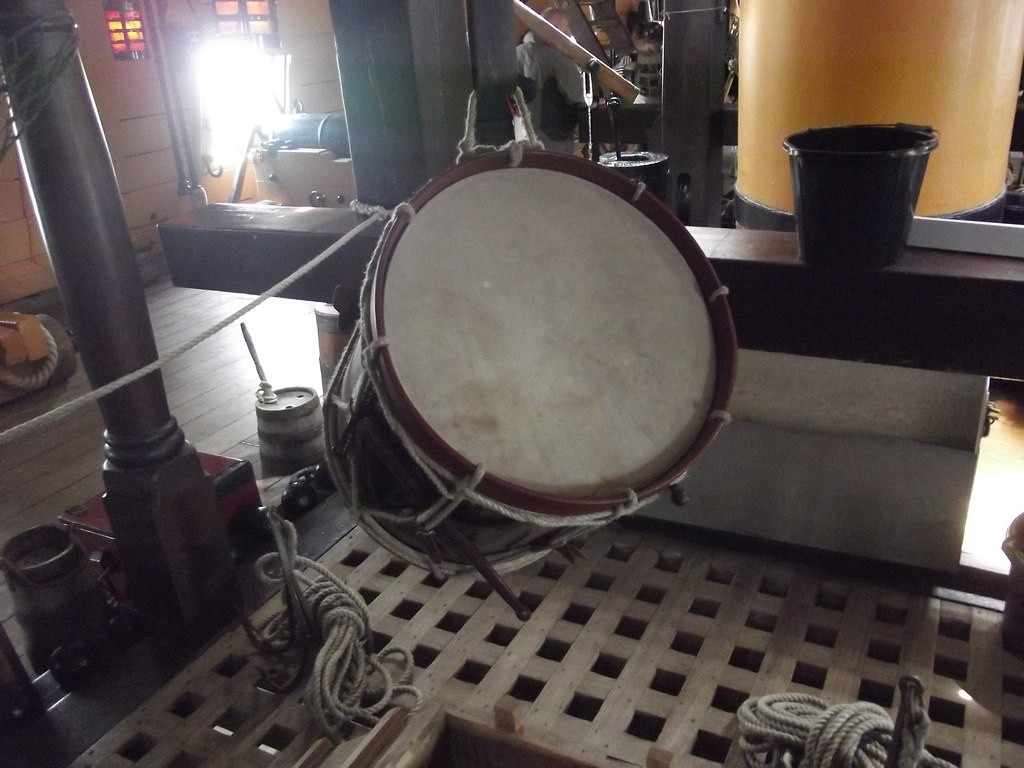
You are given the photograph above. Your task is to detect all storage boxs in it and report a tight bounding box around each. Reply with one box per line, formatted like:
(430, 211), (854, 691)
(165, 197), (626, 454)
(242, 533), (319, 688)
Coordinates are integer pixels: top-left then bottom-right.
(57, 451), (264, 605)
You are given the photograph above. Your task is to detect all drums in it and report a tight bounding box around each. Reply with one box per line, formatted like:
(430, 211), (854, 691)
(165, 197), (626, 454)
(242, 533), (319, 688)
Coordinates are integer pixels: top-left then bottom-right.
(316, 141), (741, 576)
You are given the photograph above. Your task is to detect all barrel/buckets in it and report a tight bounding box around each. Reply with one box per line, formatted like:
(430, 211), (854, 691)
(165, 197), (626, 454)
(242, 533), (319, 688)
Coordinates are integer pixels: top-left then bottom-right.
(313, 303), (345, 377)
(0, 523), (110, 644)
(256, 388), (326, 479)
(782, 122), (939, 272)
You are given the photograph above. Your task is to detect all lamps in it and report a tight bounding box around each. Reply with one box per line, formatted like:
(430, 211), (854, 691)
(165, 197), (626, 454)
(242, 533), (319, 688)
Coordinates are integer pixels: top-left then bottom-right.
(102, 0), (146, 60)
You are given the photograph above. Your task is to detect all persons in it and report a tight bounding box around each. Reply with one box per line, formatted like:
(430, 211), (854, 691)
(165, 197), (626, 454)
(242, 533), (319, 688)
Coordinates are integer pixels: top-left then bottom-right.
(610, 20), (663, 152)
(516, 6), (585, 156)
